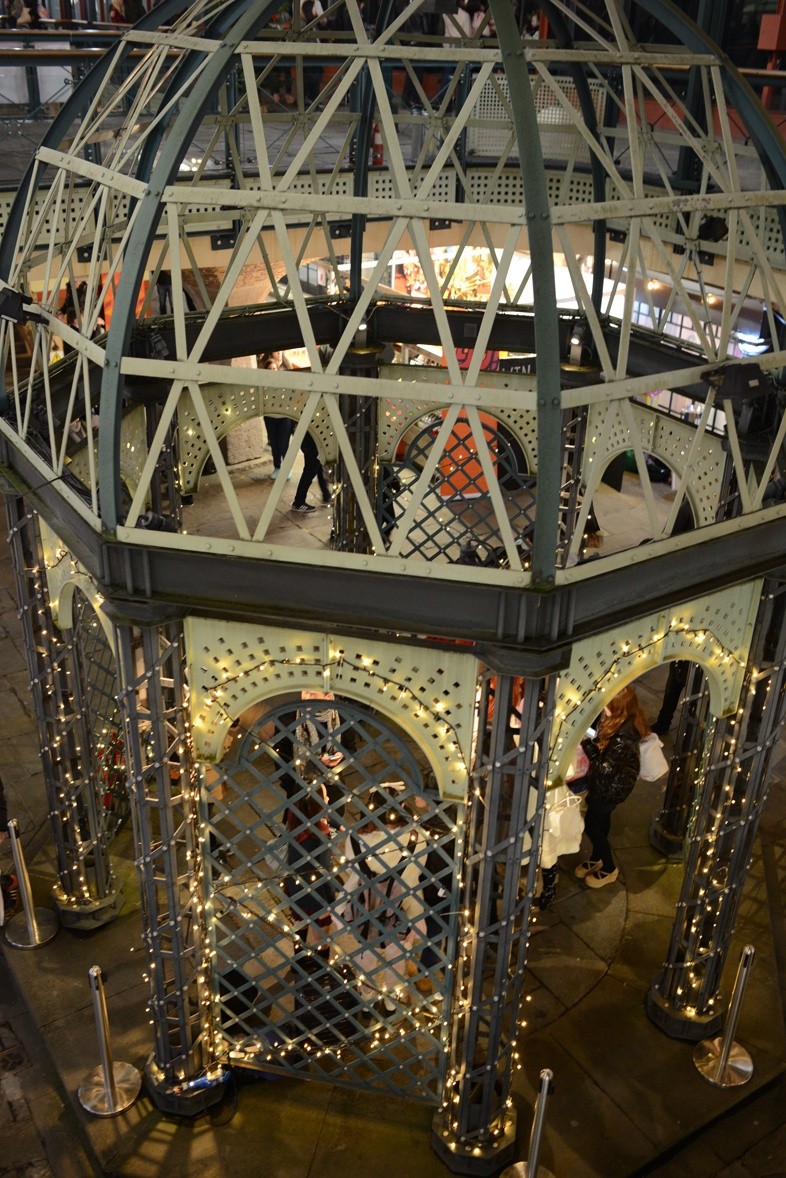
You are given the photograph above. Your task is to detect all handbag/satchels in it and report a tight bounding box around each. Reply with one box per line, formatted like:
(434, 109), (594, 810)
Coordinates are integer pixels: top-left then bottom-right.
(639, 729), (669, 782)
(564, 743), (589, 783)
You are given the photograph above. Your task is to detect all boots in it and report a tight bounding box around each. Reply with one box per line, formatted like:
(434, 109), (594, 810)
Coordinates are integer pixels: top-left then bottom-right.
(536, 867), (556, 912)
(291, 927), (309, 973)
(549, 862), (560, 901)
(381, 990), (398, 1016)
(313, 945), (330, 970)
(360, 985), (382, 1017)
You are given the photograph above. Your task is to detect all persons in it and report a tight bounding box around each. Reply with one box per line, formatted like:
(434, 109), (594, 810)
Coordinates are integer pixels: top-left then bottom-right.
(57, 268), (606, 575)
(2, 0), (541, 121)
(0, 646), (704, 1028)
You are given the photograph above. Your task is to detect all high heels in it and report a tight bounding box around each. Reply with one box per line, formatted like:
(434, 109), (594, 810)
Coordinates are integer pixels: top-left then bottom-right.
(574, 858), (603, 879)
(585, 867), (619, 889)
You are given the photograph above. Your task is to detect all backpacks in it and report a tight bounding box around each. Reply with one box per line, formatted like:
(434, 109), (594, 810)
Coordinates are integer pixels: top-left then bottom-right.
(348, 829), (418, 948)
